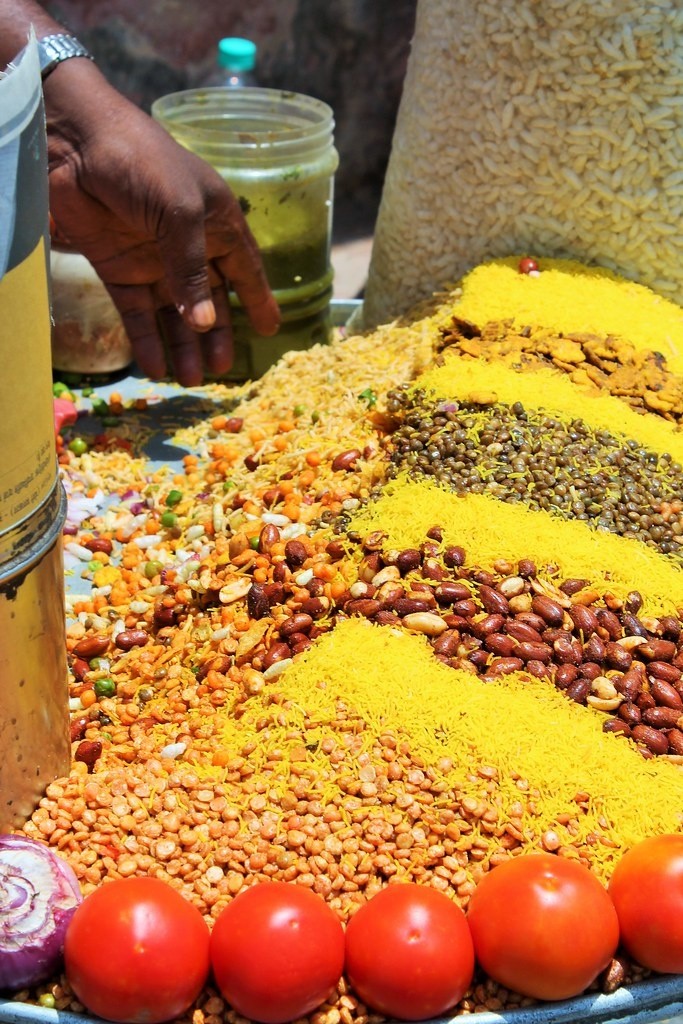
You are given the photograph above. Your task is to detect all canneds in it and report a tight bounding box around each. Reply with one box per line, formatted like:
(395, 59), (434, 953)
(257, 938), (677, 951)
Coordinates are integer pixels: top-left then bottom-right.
(151, 88), (340, 383)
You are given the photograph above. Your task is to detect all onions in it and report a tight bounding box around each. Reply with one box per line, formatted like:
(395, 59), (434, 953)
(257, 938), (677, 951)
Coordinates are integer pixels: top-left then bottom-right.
(0, 834), (82, 995)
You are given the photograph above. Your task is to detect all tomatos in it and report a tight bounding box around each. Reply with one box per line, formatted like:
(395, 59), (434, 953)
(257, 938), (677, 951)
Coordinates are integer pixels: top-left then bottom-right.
(61, 831), (683, 1022)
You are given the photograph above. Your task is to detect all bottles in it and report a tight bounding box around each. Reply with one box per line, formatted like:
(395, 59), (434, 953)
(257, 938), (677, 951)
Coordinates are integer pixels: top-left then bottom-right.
(194, 38), (261, 93)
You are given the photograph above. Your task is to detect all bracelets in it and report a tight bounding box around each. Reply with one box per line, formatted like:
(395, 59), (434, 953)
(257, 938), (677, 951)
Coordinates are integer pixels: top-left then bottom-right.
(31, 25), (94, 76)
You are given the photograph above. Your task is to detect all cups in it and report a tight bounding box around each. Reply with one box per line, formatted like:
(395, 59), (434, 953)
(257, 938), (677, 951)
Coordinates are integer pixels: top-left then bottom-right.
(1, 455), (71, 839)
(150, 86), (338, 385)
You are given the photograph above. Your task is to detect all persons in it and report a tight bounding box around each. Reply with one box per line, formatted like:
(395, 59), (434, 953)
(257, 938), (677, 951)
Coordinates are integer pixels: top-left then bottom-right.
(0, 0), (278, 381)
